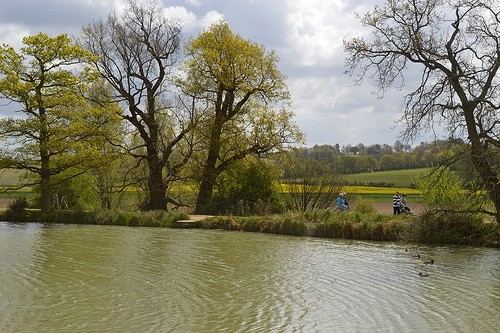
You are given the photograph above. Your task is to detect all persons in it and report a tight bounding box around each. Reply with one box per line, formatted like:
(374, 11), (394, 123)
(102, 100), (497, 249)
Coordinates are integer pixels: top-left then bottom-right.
(337, 191), (349, 211)
(392, 191), (416, 215)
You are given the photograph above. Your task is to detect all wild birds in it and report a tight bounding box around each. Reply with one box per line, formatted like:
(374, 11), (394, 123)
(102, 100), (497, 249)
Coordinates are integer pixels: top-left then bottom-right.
(423, 259), (435, 264)
(412, 254), (421, 258)
(418, 272), (429, 277)
(405, 248), (409, 252)
